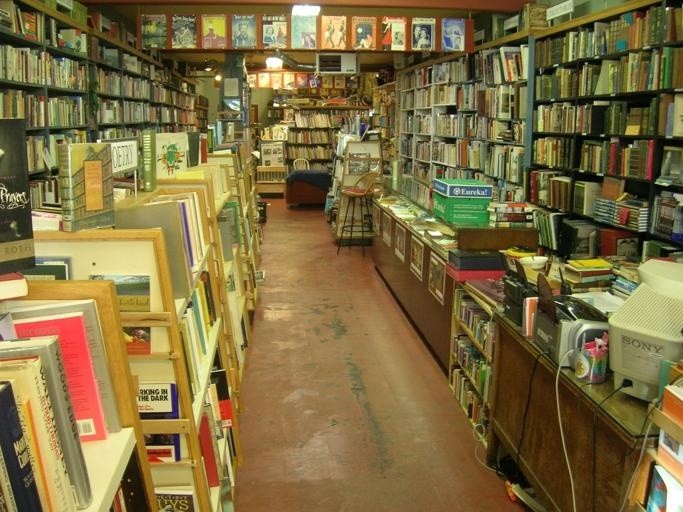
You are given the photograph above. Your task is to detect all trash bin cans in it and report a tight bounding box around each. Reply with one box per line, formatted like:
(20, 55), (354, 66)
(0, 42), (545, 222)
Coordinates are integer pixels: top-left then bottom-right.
(258, 201), (266, 222)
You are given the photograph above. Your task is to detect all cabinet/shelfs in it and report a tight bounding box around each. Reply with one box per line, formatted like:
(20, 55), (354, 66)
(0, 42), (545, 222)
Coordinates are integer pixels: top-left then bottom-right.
(253, 103), (385, 249)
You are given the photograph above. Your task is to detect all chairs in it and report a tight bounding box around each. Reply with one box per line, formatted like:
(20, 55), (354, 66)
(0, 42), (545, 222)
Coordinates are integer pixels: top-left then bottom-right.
(336, 172), (376, 259)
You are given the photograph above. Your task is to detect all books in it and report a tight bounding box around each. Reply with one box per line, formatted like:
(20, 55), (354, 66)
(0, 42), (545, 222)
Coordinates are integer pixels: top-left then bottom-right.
(1, 0), (264, 511)
(331, 1), (682, 510)
(258, 75), (341, 184)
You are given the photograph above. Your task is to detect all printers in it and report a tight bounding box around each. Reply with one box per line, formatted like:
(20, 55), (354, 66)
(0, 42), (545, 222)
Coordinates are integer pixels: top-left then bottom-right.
(503, 258), (608, 367)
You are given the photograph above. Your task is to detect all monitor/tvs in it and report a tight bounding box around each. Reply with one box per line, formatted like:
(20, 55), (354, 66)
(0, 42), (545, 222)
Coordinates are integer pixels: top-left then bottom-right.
(607, 257), (683, 403)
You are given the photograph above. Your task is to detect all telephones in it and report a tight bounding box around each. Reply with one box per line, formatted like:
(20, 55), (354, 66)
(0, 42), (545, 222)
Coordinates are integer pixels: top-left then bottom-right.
(559, 267), (572, 295)
